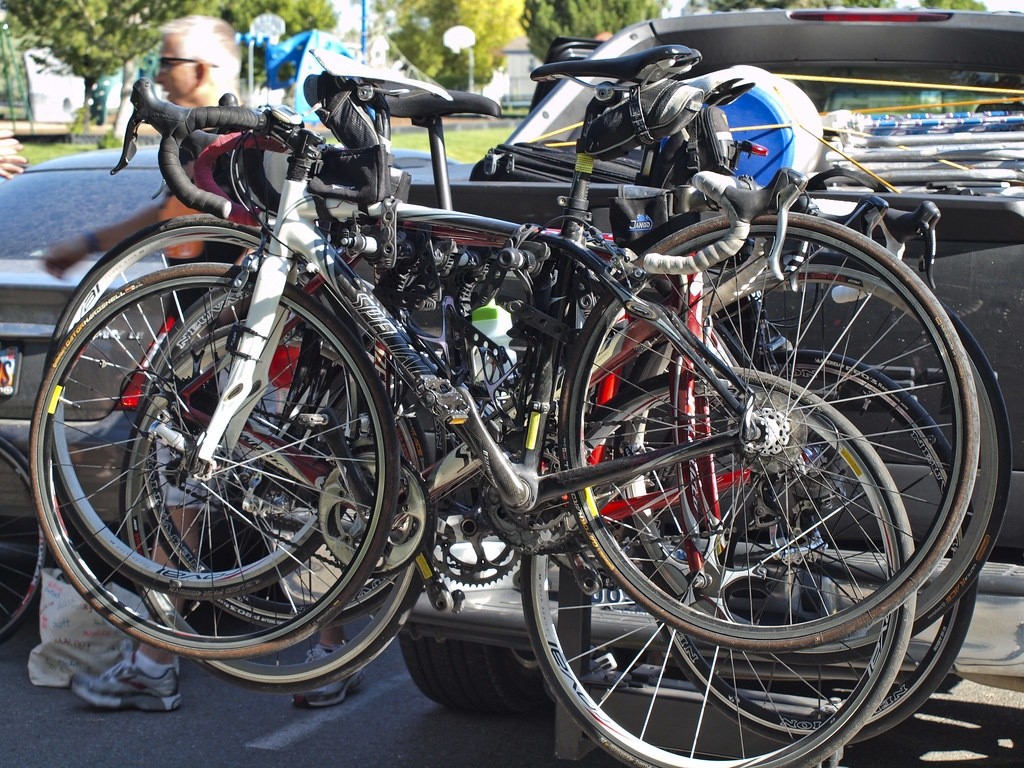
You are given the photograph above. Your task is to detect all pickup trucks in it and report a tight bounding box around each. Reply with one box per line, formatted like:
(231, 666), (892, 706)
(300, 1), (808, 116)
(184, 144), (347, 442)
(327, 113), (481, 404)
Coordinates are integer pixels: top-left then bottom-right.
(273, 7), (1024, 767)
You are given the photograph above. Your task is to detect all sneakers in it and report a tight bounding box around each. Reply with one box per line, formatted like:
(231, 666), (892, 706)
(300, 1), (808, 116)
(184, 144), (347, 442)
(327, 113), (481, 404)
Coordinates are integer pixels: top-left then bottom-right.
(70, 659), (183, 711)
(291, 639), (367, 707)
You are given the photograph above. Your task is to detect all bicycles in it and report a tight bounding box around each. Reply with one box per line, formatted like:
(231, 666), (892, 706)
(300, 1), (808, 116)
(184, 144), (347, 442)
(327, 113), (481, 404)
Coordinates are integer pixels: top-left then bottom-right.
(1, 40), (1010, 767)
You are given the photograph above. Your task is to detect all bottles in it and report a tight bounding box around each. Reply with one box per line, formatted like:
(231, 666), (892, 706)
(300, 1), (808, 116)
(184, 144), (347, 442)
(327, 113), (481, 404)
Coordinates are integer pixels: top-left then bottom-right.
(468, 295), (517, 384)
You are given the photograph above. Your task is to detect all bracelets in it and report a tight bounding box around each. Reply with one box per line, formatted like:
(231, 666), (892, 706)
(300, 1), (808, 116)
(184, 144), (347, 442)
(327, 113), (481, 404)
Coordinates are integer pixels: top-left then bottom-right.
(84, 230), (103, 253)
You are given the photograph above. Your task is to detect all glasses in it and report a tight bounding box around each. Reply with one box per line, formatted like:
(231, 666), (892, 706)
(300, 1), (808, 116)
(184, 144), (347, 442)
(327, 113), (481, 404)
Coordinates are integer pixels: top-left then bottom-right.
(159, 56), (220, 69)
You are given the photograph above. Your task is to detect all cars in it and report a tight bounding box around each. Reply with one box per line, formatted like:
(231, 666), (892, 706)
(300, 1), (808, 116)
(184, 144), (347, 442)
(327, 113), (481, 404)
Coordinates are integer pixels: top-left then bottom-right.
(2, 140), (471, 530)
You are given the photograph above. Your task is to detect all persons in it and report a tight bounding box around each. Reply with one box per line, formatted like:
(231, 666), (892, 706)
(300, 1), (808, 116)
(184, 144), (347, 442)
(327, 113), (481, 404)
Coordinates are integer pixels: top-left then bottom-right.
(0, 129), (28, 178)
(39, 15), (364, 709)
